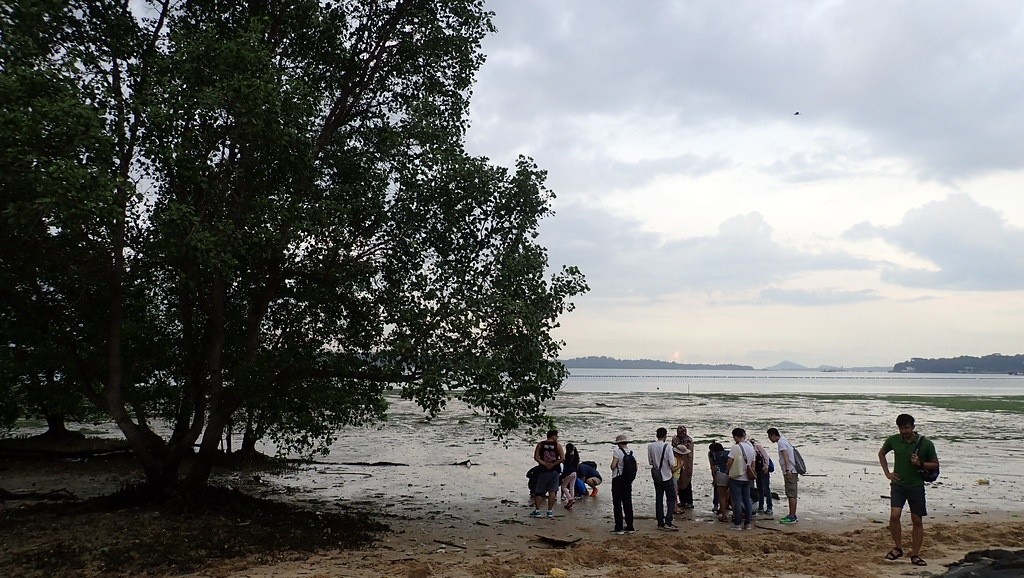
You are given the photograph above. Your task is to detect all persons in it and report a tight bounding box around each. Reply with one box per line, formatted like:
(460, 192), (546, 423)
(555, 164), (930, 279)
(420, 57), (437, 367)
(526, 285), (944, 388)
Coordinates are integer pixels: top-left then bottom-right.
(725, 428), (753, 530)
(673, 445), (690, 515)
(530, 430), (565, 517)
(673, 425), (694, 508)
(708, 442), (733, 522)
(742, 429), (775, 520)
(526, 465), (538, 494)
(767, 428), (798, 524)
(562, 443), (579, 508)
(555, 462), (602, 500)
(648, 428), (678, 531)
(879, 414), (939, 566)
(611, 435), (635, 534)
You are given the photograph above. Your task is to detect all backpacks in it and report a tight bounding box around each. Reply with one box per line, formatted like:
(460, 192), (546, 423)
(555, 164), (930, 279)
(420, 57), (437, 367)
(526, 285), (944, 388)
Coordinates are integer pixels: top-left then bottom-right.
(617, 447), (637, 485)
(913, 434), (939, 482)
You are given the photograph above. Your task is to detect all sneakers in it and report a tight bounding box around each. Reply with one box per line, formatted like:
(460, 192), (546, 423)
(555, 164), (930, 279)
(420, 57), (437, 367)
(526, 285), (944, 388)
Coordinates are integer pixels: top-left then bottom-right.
(781, 516), (798, 522)
(780, 517), (796, 523)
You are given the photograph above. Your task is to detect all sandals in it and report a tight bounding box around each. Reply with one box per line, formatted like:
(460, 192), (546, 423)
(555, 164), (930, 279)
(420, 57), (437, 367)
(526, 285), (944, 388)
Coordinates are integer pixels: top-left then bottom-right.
(887, 547), (904, 560)
(911, 555), (928, 566)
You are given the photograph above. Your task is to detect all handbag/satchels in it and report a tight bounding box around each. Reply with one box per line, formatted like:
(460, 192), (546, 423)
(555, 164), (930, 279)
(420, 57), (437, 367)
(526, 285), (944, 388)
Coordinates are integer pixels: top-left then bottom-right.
(651, 467), (663, 484)
(715, 471), (728, 487)
(762, 456), (774, 472)
(793, 449), (806, 475)
(747, 465), (757, 479)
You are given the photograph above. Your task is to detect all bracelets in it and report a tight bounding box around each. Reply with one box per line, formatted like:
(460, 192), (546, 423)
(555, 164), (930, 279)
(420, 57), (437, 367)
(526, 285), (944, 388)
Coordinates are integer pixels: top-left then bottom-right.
(920, 461), (924, 466)
(786, 470), (789, 472)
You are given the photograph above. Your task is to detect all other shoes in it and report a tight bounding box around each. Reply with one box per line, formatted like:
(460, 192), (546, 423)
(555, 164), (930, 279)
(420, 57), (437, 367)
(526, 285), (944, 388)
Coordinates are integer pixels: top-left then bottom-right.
(563, 505), (574, 510)
(547, 511), (554, 517)
(590, 489), (598, 496)
(663, 524), (680, 530)
(726, 523), (741, 531)
(623, 526), (635, 533)
(529, 510), (541, 517)
(566, 499), (575, 508)
(610, 529), (625, 535)
(715, 508), (773, 524)
(744, 523), (752, 529)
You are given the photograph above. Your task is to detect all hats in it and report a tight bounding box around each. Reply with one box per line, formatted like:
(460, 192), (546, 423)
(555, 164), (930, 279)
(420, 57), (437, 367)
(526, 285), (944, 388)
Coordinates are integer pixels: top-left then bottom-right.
(613, 434), (633, 444)
(672, 444), (692, 454)
